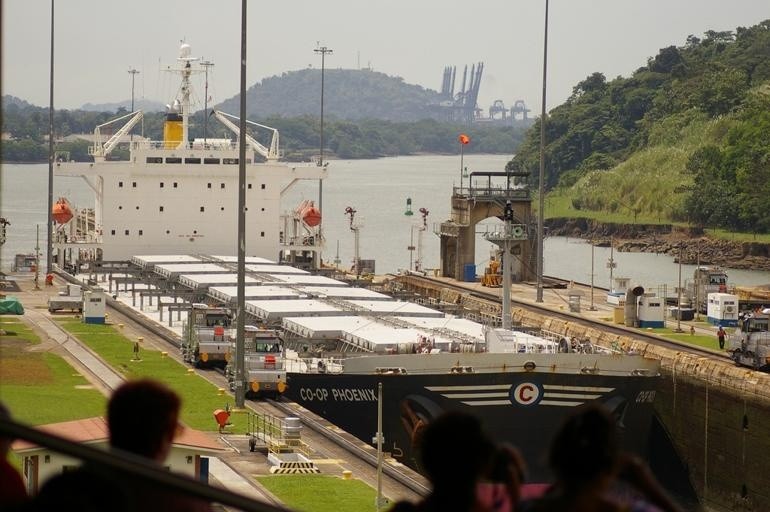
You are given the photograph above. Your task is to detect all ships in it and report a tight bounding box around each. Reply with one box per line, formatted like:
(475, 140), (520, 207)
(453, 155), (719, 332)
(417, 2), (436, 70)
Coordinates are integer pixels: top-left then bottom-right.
(49, 39), (661, 512)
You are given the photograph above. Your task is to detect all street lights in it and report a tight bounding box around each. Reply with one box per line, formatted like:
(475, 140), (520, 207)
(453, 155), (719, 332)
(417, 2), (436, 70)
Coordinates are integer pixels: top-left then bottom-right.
(585, 237), (598, 312)
(312, 46), (333, 236)
(200, 57), (214, 141)
(126, 66), (141, 144)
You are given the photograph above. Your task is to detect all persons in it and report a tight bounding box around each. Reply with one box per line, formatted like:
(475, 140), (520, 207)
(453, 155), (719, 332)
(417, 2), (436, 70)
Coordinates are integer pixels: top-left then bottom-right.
(29, 379), (210, 512)
(0, 403), (28, 512)
(710, 278), (718, 283)
(717, 325), (727, 348)
(519, 402), (685, 511)
(719, 280), (727, 293)
(386, 411), (528, 512)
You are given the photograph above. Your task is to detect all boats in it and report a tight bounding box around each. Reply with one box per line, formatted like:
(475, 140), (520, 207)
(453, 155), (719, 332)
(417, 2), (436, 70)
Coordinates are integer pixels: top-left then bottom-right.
(12, 252), (40, 273)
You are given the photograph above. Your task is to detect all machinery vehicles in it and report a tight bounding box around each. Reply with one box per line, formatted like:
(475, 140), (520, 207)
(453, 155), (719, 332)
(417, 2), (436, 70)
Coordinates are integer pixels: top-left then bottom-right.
(181, 299), (234, 366)
(727, 308), (770, 370)
(482, 248), (502, 287)
(674, 266), (730, 313)
(226, 324), (288, 400)
